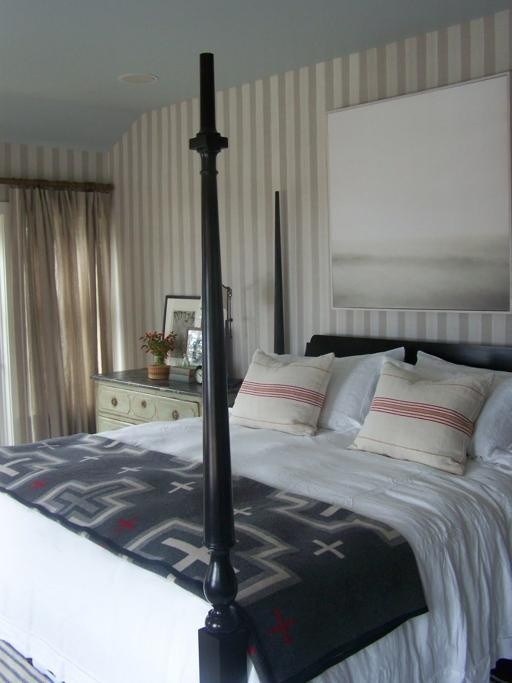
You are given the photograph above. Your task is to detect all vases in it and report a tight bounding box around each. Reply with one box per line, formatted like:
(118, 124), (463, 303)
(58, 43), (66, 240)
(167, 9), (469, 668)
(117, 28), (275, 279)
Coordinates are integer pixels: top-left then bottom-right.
(146, 350), (171, 379)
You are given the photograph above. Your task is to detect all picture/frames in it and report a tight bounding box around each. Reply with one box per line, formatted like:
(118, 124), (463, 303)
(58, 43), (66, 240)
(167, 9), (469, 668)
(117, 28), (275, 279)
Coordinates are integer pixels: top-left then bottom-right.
(323, 69), (510, 315)
(160, 293), (204, 367)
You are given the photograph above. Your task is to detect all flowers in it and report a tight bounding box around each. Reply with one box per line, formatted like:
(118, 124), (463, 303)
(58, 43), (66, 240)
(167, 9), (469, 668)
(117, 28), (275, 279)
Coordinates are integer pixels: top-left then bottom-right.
(139, 329), (177, 357)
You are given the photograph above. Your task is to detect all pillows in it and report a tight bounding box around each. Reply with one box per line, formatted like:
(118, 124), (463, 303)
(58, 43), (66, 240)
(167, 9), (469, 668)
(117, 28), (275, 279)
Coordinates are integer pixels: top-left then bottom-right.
(228, 347), (510, 479)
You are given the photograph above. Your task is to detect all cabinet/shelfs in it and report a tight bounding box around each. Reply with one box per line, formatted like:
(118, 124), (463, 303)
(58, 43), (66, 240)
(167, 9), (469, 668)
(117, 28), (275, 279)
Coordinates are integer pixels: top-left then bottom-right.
(89, 366), (243, 432)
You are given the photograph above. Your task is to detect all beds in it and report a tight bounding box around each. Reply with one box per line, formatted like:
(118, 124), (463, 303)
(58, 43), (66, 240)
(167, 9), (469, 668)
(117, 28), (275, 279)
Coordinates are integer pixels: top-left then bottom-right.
(0, 334), (511, 683)
